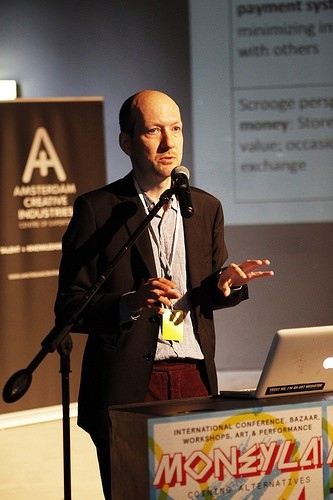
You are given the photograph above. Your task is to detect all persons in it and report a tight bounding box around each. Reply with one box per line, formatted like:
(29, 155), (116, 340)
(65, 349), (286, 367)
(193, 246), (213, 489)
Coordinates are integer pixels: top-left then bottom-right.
(52, 88), (276, 500)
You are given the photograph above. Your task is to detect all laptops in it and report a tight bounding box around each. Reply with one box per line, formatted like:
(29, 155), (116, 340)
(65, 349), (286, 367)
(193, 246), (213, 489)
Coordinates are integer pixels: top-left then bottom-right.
(219, 325), (333, 398)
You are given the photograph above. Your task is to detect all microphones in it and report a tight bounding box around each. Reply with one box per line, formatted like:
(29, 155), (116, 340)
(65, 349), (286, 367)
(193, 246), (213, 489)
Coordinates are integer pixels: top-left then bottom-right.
(171, 166), (194, 218)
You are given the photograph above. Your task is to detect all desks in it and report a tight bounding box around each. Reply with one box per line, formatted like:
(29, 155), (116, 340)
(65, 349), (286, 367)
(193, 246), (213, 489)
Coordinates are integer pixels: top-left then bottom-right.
(108, 392), (333, 500)
